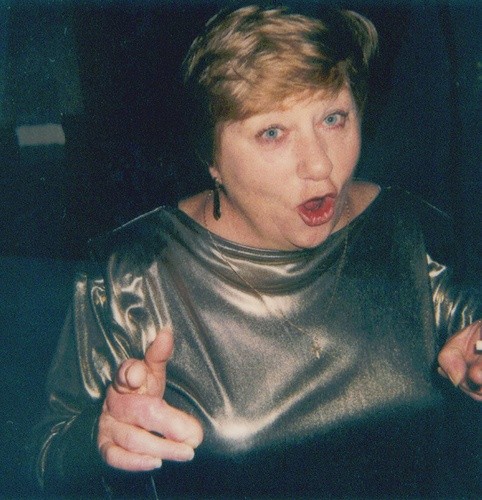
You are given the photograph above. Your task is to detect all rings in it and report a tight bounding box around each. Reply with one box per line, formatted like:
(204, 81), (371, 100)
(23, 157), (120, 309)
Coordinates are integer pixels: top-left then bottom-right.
(473, 339), (481, 355)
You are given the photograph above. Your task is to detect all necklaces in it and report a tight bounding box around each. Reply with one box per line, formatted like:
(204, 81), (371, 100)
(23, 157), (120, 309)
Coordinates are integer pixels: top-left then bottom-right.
(202, 185), (352, 363)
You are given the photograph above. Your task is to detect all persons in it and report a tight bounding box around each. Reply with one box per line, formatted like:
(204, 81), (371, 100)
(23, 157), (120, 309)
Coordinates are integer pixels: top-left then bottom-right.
(26, 0), (481, 500)
